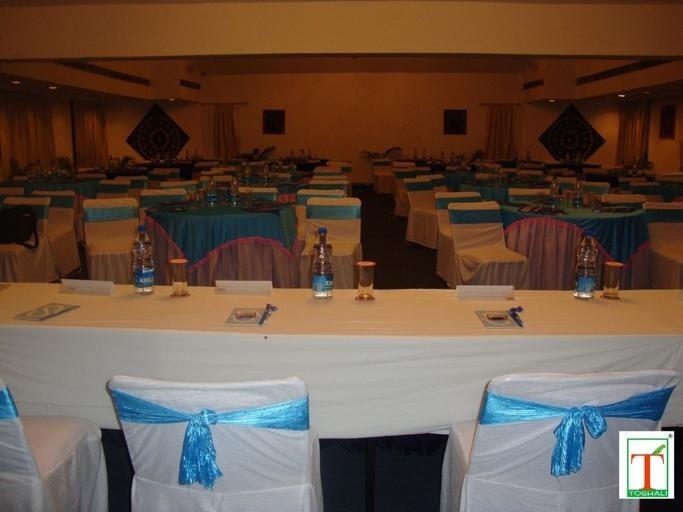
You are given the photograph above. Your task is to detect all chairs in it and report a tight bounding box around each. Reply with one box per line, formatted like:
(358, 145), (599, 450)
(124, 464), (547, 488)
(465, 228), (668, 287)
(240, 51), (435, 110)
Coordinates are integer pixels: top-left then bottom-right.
(0, 144), (683, 511)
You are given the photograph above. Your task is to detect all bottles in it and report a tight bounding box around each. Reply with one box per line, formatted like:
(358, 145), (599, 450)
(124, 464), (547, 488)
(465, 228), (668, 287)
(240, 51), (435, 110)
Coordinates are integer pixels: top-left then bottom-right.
(243, 163), (251, 187)
(573, 227), (599, 301)
(129, 224), (155, 296)
(229, 175), (241, 208)
(412, 147), (530, 186)
(617, 159), (638, 178)
(311, 227), (335, 301)
(30, 147), (198, 186)
(549, 176), (560, 209)
(205, 173), (218, 210)
(263, 163), (272, 187)
(575, 153), (581, 167)
(573, 175), (583, 210)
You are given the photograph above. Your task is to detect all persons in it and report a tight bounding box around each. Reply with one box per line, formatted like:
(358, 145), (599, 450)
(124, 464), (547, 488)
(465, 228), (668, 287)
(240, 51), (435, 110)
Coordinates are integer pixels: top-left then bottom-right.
(560, 152), (573, 169)
(573, 152), (584, 180)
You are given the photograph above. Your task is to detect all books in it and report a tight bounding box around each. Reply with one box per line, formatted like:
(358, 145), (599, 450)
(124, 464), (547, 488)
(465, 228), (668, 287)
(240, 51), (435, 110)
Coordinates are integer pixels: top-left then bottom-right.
(222, 307), (270, 327)
(12, 303), (80, 321)
(474, 310), (527, 330)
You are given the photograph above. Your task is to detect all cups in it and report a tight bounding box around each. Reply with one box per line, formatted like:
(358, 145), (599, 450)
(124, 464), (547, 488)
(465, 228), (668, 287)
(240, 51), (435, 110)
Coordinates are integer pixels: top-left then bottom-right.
(601, 260), (623, 300)
(168, 258), (188, 297)
(587, 191), (603, 214)
(351, 261), (375, 301)
(566, 191), (573, 208)
(240, 188), (254, 208)
